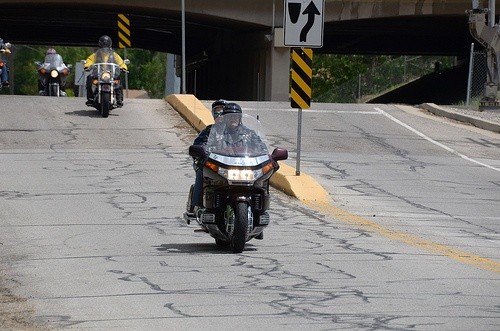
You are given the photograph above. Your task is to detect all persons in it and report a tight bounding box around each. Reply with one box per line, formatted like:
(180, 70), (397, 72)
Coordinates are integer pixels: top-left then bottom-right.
(39, 47), (67, 97)
(83, 35), (129, 107)
(189, 99), (271, 239)
(0, 38), (12, 88)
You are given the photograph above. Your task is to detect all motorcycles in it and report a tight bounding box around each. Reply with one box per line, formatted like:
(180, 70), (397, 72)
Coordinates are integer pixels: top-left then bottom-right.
(81, 45), (130, 118)
(0, 38), (11, 95)
(182, 112), (289, 254)
(34, 53), (72, 97)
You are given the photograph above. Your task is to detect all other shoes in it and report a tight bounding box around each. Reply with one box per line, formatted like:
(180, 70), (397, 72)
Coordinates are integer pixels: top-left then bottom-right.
(85, 99), (94, 105)
(117, 100), (123, 107)
(39, 90), (44, 94)
(60, 89), (66, 96)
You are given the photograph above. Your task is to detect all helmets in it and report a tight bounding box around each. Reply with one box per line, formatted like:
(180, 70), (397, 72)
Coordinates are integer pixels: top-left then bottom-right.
(98, 35), (112, 48)
(220, 103), (243, 115)
(47, 48), (57, 54)
(211, 100), (229, 118)
